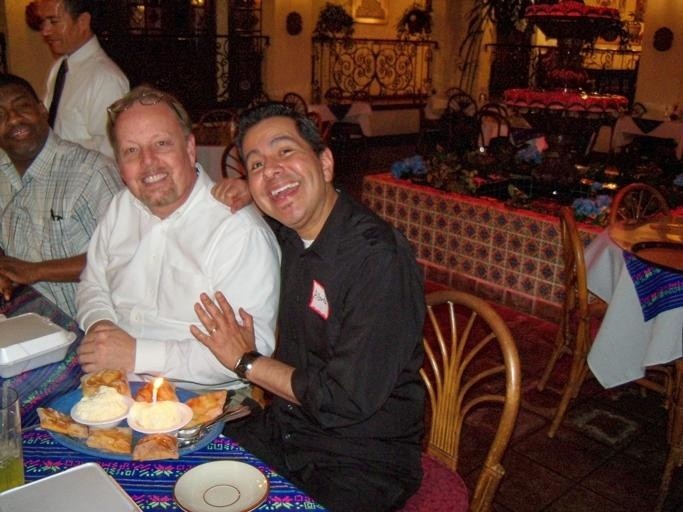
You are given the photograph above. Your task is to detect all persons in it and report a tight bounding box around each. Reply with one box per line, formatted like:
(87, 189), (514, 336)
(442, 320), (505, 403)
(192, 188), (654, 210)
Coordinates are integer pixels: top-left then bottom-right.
(191, 102), (427, 512)
(32, 0), (129, 159)
(77, 86), (281, 396)
(0, 71), (125, 316)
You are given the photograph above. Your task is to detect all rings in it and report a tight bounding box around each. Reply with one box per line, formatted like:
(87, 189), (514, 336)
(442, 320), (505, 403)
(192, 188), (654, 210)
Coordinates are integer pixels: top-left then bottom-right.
(209, 326), (218, 336)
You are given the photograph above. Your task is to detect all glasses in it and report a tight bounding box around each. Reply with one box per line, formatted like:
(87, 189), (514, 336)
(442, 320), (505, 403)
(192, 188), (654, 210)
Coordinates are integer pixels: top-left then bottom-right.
(105, 86), (176, 126)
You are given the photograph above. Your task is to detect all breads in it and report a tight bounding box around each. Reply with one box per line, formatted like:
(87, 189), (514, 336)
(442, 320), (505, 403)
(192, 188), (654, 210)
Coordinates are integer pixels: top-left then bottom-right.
(135, 432), (179, 460)
(184, 388), (226, 428)
(35, 406), (90, 440)
(137, 377), (180, 402)
(82, 421), (131, 455)
(80, 367), (130, 397)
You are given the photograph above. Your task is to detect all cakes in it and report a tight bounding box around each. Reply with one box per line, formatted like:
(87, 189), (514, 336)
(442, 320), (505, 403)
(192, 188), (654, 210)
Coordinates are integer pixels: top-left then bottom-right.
(75, 385), (127, 422)
(133, 400), (184, 432)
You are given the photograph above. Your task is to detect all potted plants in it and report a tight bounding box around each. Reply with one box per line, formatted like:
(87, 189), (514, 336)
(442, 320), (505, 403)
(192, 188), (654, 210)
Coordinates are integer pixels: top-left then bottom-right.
(313, 2), (357, 51)
(394, 2), (433, 43)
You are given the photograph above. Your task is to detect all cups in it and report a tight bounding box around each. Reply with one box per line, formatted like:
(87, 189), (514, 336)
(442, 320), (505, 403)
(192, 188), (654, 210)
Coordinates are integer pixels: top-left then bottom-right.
(1, 386), (26, 491)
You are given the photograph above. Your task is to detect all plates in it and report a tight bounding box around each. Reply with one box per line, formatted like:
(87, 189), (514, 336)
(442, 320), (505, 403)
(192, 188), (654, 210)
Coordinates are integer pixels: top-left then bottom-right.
(173, 460), (270, 512)
(45, 381), (228, 462)
(128, 401), (193, 435)
(70, 395), (134, 430)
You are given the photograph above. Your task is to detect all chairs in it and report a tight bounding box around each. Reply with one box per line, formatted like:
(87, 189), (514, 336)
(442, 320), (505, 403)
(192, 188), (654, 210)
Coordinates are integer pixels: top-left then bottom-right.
(221, 137), (248, 182)
(244, 88), (270, 106)
(447, 92), (478, 122)
(198, 108), (239, 127)
(446, 86), (464, 99)
(536, 204), (683, 441)
(396, 289), (522, 512)
(282, 92), (310, 121)
(609, 182), (670, 226)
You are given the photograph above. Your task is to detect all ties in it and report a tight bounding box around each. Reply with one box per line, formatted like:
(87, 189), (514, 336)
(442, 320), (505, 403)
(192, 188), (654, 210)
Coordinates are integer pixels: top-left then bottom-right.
(46, 58), (69, 127)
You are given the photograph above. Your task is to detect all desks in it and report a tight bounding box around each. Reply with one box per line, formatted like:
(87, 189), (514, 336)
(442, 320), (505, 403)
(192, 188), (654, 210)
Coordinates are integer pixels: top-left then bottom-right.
(359, 151), (609, 339)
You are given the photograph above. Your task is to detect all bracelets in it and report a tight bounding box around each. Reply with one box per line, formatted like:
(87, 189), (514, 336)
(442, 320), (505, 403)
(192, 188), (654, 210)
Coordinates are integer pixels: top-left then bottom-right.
(234, 350), (262, 378)
